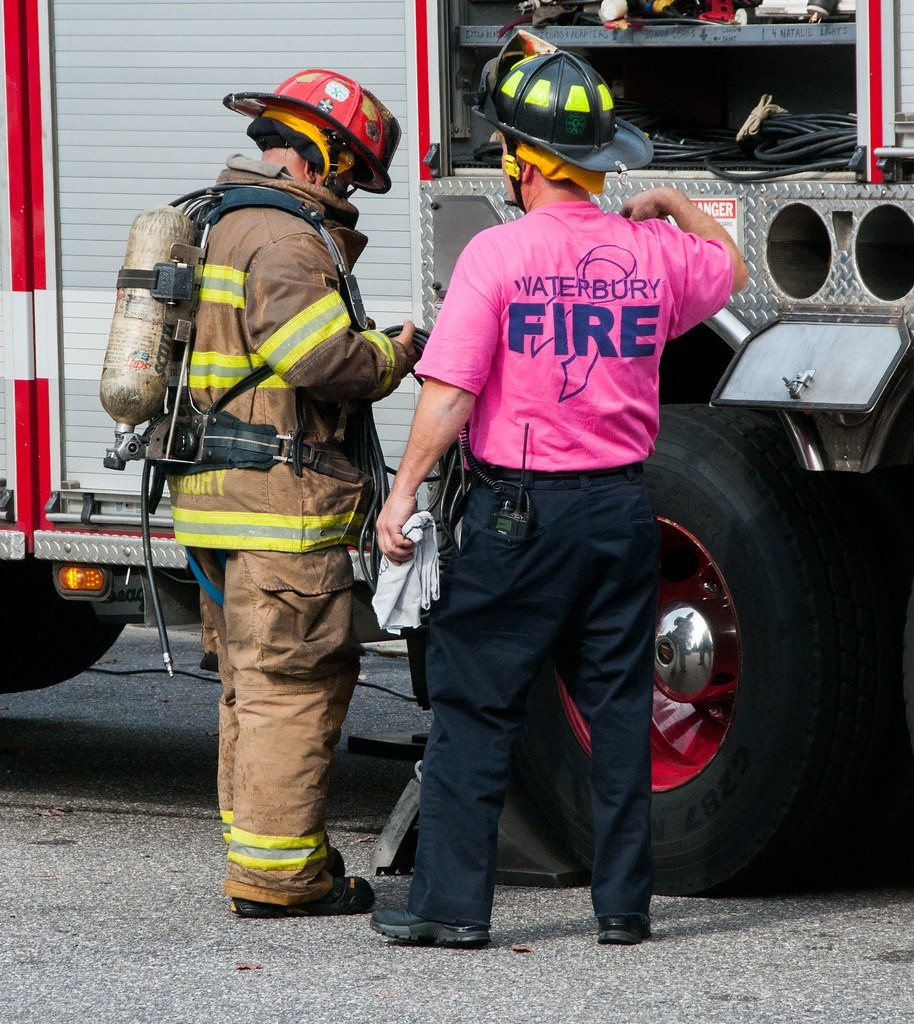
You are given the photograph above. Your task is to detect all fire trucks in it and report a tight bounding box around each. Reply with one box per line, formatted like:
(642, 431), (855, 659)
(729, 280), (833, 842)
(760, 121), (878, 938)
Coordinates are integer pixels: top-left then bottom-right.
(0, 0), (911, 894)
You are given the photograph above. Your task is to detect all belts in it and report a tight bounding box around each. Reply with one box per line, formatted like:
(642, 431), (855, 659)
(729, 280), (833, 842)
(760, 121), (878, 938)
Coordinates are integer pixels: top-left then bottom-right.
(480, 462), (647, 480)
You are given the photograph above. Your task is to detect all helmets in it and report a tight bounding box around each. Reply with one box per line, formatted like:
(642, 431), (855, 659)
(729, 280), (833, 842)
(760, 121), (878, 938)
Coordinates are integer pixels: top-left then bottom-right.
(225, 68), (400, 194)
(468, 31), (653, 170)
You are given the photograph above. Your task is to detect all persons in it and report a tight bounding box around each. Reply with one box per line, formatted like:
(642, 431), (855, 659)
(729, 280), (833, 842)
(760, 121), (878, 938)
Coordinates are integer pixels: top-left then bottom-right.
(165, 69), (420, 921)
(368, 51), (749, 948)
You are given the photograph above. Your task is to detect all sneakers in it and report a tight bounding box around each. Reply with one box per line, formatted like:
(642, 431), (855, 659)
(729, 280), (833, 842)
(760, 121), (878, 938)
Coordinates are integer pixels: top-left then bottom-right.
(321, 843), (346, 876)
(370, 905), (491, 947)
(599, 911), (652, 944)
(231, 873), (375, 919)
